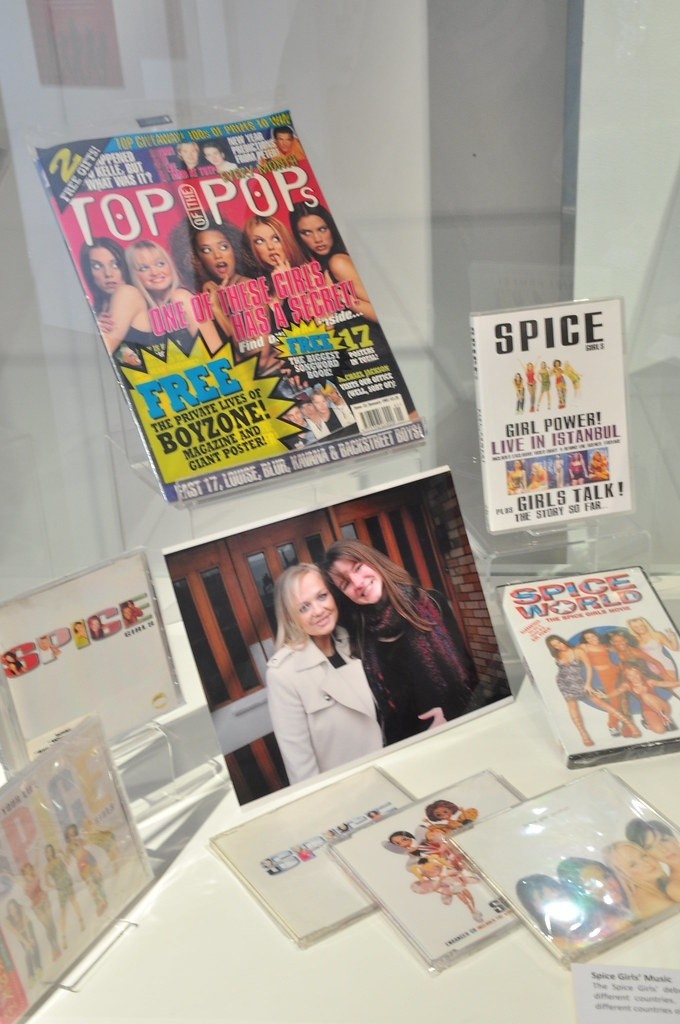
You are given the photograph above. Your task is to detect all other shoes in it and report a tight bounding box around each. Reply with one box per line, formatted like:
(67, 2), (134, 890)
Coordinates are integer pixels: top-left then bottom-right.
(622, 729), (633, 737)
(608, 727), (620, 735)
(632, 730), (641, 737)
(583, 738), (594, 746)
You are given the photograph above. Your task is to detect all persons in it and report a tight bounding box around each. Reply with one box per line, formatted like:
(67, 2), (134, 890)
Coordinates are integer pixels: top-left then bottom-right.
(78, 200), (416, 451)
(320, 538), (488, 745)
(176, 141), (201, 178)
(71, 615), (104, 650)
(36, 636), (60, 663)
(260, 809), (381, 874)
(120, 600), (144, 628)
(507, 450), (609, 495)
(202, 140), (237, 173)
(514, 358), (582, 415)
(259, 125), (305, 168)
(4, 818), (120, 988)
(265, 562), (384, 787)
(4, 653), (28, 675)
(515, 816), (680, 955)
(545, 618), (680, 745)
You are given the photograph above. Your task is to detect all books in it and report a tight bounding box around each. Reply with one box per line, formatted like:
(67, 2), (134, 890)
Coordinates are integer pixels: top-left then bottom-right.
(469, 297), (634, 534)
(495, 564), (680, 769)
(32, 107), (427, 506)
